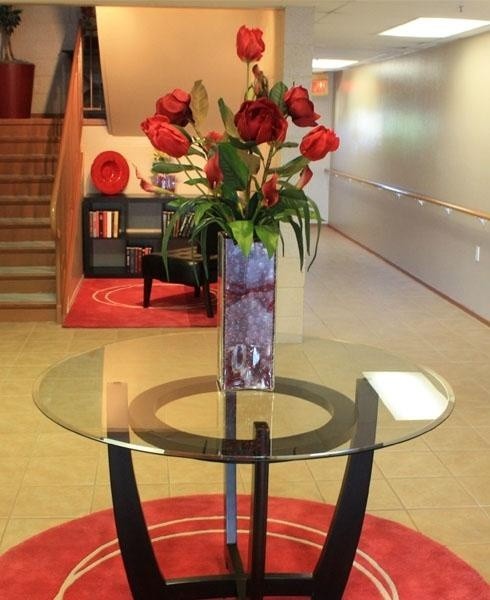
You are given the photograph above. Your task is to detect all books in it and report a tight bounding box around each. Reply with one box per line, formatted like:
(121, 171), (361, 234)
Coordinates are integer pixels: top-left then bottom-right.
(88, 210), (120, 239)
(125, 247), (152, 274)
(162, 210), (195, 238)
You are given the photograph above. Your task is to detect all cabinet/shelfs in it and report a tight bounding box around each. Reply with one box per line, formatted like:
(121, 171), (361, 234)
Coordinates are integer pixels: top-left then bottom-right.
(81, 193), (213, 278)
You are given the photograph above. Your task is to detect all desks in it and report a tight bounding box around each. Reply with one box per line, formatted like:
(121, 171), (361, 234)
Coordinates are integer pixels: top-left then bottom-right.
(31, 331), (455, 600)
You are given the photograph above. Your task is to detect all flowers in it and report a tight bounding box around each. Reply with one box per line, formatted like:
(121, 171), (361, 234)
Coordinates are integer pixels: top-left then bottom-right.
(132, 23), (341, 285)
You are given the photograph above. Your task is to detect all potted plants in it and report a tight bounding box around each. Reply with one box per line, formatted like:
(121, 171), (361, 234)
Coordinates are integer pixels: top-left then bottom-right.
(0, 5), (35, 118)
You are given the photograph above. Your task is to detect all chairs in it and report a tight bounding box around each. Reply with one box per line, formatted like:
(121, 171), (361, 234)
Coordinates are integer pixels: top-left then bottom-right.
(141, 213), (221, 317)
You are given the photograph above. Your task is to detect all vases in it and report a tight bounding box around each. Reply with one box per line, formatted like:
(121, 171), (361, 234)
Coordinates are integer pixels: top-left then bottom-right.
(217, 233), (277, 393)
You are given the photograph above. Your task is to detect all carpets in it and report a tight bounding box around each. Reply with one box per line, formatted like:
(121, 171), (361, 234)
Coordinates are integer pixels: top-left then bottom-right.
(62, 277), (221, 327)
(0, 495), (490, 600)
